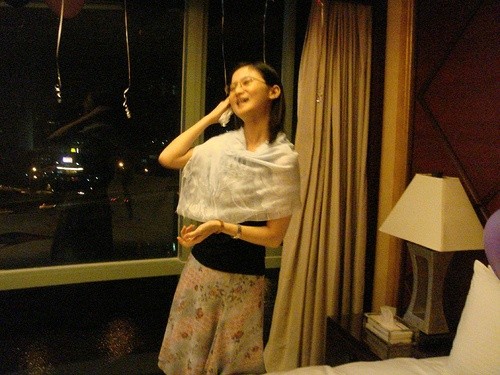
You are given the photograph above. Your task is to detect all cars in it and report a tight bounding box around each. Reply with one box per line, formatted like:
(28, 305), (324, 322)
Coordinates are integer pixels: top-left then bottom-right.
(105, 187), (131, 206)
(13, 194), (56, 210)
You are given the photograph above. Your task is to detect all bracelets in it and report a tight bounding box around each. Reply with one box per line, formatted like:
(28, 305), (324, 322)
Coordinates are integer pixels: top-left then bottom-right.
(214, 218), (224, 236)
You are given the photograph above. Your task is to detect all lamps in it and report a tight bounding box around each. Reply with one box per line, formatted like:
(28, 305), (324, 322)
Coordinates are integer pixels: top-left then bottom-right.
(378, 169), (487, 335)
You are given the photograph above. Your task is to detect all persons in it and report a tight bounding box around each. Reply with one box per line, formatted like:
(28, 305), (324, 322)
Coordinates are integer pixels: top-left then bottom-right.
(158, 61), (303, 375)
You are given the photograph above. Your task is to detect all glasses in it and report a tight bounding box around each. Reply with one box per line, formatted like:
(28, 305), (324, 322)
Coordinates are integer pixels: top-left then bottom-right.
(225, 76), (267, 91)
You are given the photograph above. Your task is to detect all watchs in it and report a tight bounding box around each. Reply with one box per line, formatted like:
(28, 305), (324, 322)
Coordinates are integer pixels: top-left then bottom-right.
(232, 222), (243, 240)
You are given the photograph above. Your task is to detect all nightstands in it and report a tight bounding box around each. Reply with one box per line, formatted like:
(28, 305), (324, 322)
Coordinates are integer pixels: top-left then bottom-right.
(326, 310), (460, 370)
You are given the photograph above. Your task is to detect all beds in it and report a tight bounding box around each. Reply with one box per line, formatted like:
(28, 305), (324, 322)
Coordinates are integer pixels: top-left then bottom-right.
(265, 354), (450, 375)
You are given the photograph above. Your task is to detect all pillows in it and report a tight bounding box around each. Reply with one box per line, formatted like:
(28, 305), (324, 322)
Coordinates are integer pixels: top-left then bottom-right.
(445, 258), (500, 375)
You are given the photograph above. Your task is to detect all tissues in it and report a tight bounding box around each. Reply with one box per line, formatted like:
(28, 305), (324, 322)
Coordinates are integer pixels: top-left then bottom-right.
(362, 305), (419, 349)
